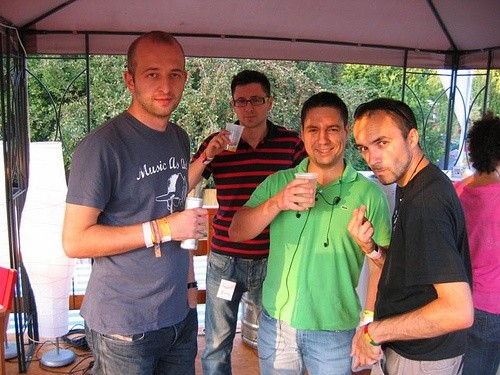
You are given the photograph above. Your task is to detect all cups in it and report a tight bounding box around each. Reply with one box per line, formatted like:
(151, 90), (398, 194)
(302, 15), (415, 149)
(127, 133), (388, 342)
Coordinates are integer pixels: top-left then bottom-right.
(222, 122), (246, 152)
(294, 172), (318, 208)
(180, 196), (203, 250)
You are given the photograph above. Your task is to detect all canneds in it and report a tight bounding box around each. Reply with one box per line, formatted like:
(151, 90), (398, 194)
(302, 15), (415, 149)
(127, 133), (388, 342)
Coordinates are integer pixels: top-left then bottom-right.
(180, 197), (203, 250)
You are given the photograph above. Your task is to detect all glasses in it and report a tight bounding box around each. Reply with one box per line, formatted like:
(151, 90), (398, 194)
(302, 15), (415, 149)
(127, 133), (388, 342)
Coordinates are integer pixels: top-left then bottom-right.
(233, 96), (269, 107)
(354, 100), (412, 124)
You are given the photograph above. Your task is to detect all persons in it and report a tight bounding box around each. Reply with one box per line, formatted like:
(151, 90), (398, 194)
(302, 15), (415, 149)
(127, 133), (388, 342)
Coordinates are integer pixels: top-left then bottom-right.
(185, 69), (310, 375)
(346, 96), (474, 375)
(451, 110), (500, 375)
(62, 30), (209, 375)
(228, 92), (390, 375)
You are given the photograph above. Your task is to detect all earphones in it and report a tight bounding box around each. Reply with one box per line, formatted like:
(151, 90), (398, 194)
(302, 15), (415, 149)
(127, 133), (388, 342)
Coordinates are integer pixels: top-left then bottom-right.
(295, 210), (301, 218)
(324, 239), (330, 248)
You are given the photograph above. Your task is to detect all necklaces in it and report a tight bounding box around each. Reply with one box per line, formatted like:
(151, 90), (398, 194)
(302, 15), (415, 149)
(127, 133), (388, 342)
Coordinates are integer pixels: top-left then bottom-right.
(393, 153), (425, 232)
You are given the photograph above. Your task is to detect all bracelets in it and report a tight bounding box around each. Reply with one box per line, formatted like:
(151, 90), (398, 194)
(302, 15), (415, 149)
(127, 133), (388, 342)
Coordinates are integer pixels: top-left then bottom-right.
(143, 218), (172, 258)
(200, 151), (212, 164)
(188, 281), (198, 288)
(366, 245), (382, 261)
(360, 311), (375, 326)
(364, 322), (381, 346)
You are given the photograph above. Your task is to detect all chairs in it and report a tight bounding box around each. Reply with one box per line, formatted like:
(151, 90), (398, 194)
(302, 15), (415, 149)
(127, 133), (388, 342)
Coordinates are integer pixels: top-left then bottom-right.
(0, 266), (18, 375)
